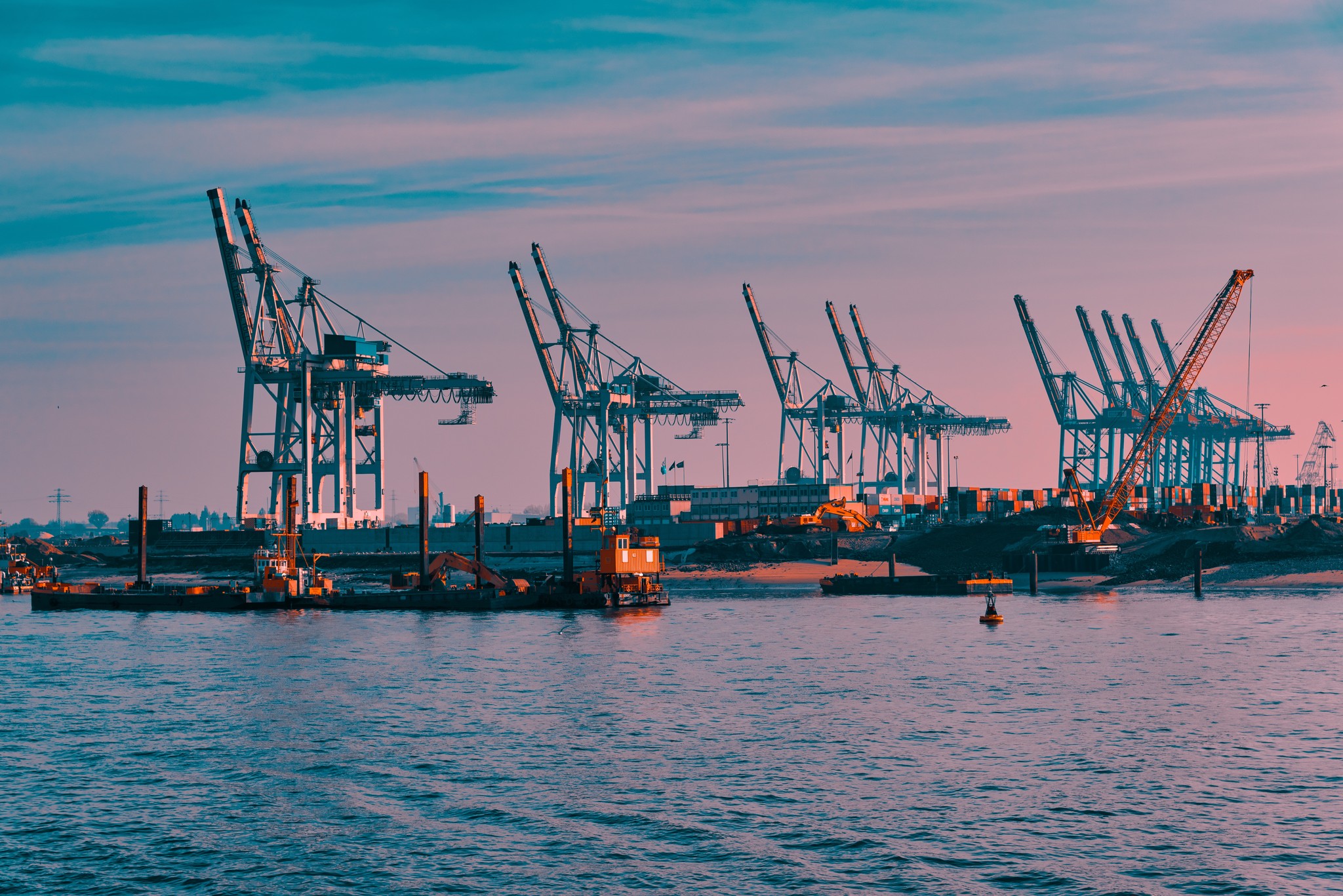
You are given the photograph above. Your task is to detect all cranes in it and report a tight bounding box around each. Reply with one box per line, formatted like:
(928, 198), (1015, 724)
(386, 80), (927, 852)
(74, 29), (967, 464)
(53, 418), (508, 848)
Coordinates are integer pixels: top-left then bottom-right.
(1014, 268), (1294, 541)
(200, 188), (497, 528)
(413, 456), (444, 515)
(508, 240), (743, 512)
(740, 275), (1012, 496)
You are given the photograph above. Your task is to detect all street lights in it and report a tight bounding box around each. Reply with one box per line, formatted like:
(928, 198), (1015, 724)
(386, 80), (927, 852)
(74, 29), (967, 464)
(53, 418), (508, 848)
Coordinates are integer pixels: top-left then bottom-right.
(714, 443), (730, 488)
(1293, 454), (1303, 485)
(954, 456), (959, 519)
(807, 427), (821, 484)
(1325, 479), (1331, 515)
(942, 435), (955, 487)
(718, 418), (736, 488)
(1326, 462), (1339, 490)
(1273, 465), (1278, 514)
(1253, 403), (1271, 488)
(569, 401), (583, 518)
(928, 427), (946, 520)
(1248, 431), (1267, 523)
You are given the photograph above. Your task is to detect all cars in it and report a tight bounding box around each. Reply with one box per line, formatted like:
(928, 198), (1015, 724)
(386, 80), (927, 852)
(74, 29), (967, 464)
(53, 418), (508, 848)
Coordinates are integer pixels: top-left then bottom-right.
(883, 526), (898, 532)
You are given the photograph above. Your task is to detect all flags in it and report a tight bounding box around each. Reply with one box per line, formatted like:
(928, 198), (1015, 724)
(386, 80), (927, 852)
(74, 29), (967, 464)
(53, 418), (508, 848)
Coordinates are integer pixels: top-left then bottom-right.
(856, 470), (863, 476)
(668, 461), (675, 471)
(846, 453), (852, 465)
(661, 458), (666, 475)
(676, 461), (684, 468)
(272, 538), (277, 546)
(602, 476), (608, 486)
(290, 500), (299, 507)
(821, 453), (829, 460)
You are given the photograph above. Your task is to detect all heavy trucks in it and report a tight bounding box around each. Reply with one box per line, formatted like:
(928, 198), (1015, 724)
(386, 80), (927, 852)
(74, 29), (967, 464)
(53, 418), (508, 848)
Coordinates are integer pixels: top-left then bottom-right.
(758, 515), (786, 526)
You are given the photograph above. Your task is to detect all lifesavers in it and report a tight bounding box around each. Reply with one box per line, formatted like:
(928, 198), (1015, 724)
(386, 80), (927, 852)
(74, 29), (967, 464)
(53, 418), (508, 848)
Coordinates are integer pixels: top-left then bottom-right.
(112, 599), (119, 609)
(176, 597), (182, 605)
(552, 601), (561, 608)
(583, 598), (589, 607)
(49, 597), (59, 606)
(589, 596), (598, 606)
(566, 598), (574, 606)
(542, 596), (551, 606)
(599, 596), (607, 606)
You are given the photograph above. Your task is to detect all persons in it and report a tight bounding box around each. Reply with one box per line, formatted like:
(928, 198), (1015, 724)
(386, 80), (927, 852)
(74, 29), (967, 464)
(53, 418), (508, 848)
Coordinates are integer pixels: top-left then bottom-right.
(465, 585), (470, 589)
(451, 586), (455, 590)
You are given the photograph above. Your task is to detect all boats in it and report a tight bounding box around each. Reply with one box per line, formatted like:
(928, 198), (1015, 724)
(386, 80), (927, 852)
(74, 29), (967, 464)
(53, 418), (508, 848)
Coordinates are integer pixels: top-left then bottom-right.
(979, 577), (1004, 622)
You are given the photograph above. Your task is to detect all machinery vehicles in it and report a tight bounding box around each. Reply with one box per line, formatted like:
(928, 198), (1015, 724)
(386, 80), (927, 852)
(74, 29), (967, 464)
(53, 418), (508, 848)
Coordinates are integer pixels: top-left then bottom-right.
(786, 496), (884, 533)
(389, 549), (530, 596)
